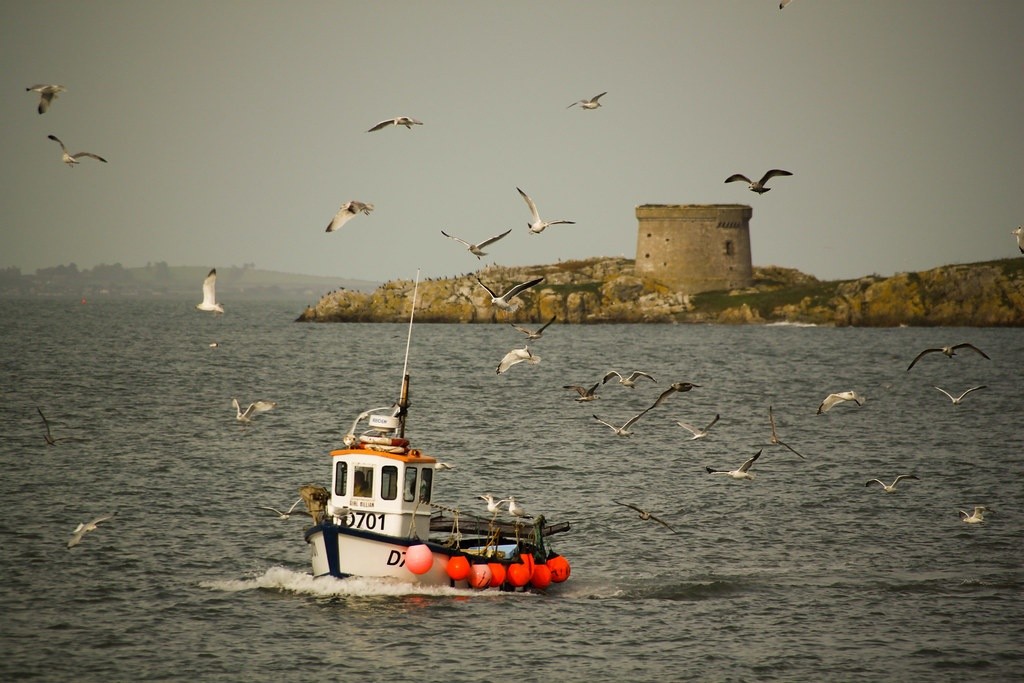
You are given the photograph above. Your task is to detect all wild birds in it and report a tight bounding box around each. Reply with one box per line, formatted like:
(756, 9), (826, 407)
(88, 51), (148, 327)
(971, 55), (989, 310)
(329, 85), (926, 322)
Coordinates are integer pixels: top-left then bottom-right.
(324, 113), (426, 236)
(611, 497), (681, 538)
(475, 493), (526, 520)
(906, 337), (992, 373)
(769, 405), (808, 459)
(494, 345), (541, 376)
(566, 91), (608, 110)
(707, 447), (763, 481)
(591, 411), (646, 439)
(866, 472), (999, 524)
(648, 382), (704, 413)
(817, 390), (866, 418)
(36, 267), (358, 551)
(933, 385), (989, 405)
(441, 177), (585, 312)
(727, 169), (793, 195)
(677, 413), (720, 440)
(512, 315), (558, 341)
(602, 370), (658, 389)
(25, 83), (110, 170)
(435, 462), (454, 472)
(562, 382), (601, 401)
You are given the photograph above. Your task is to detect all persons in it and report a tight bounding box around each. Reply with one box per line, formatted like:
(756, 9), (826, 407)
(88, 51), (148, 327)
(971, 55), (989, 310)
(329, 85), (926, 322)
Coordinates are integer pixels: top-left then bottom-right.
(354, 471), (372, 497)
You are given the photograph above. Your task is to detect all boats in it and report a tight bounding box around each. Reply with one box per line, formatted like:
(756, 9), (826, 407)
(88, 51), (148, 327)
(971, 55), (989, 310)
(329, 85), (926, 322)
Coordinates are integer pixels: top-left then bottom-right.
(303, 268), (573, 594)
